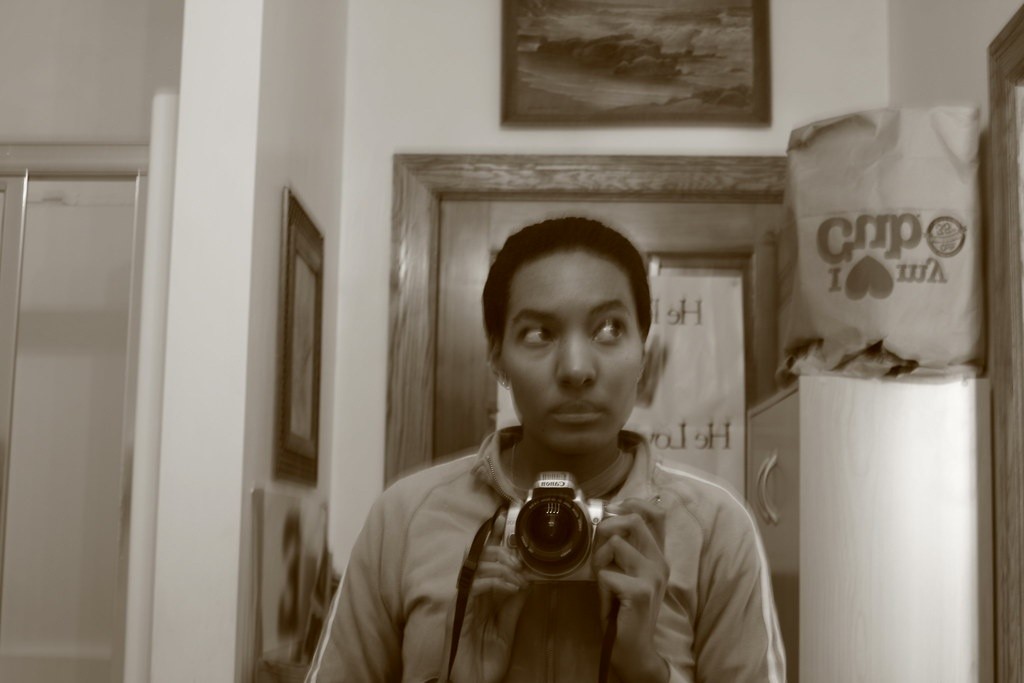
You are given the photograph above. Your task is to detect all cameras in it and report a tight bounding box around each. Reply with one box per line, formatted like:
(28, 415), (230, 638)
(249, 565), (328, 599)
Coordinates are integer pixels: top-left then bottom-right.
(501, 472), (626, 581)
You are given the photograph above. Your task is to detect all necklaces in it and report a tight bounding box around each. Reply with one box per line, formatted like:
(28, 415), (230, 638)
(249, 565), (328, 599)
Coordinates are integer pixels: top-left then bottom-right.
(511, 443), (516, 484)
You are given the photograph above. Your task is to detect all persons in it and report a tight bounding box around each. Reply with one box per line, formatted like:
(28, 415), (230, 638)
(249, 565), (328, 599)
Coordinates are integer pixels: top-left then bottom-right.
(277, 503), (330, 667)
(304, 215), (786, 683)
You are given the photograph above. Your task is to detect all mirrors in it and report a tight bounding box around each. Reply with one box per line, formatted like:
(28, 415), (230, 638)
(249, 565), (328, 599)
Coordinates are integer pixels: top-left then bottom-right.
(497, 0), (770, 131)
(0, 0), (1024, 683)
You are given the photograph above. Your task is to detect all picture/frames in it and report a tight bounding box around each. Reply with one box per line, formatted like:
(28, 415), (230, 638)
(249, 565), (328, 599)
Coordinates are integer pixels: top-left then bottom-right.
(250, 482), (333, 659)
(272, 188), (325, 484)
(383, 149), (797, 497)
